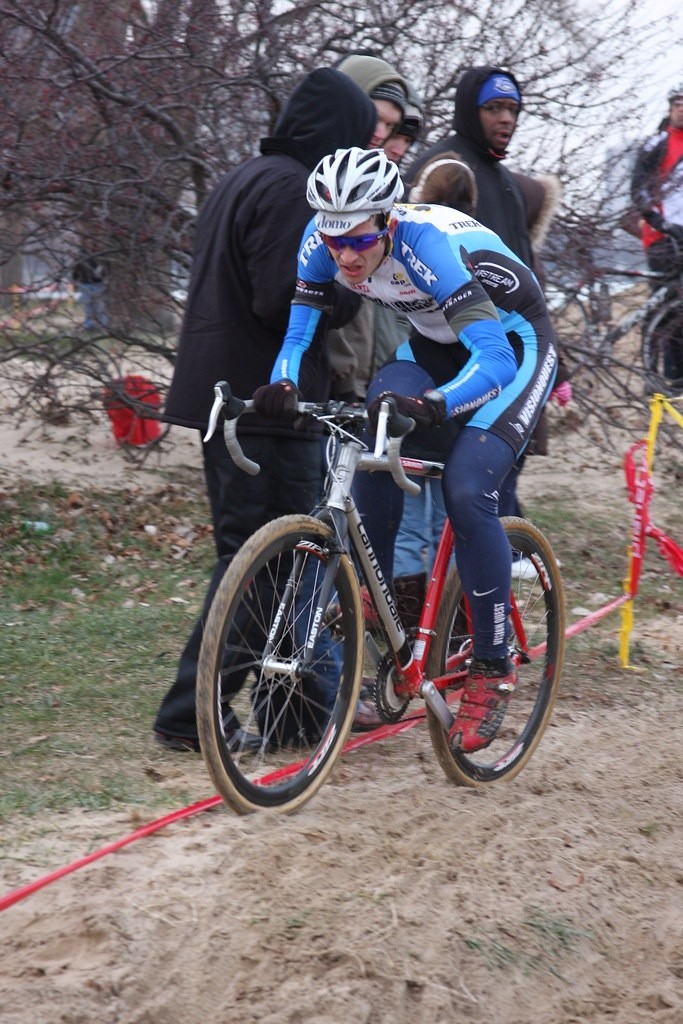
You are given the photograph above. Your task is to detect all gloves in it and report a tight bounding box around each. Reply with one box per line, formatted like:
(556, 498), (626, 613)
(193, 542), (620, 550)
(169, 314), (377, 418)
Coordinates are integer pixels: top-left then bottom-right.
(643, 211), (683, 240)
(547, 380), (572, 406)
(253, 378), (305, 422)
(368, 389), (446, 437)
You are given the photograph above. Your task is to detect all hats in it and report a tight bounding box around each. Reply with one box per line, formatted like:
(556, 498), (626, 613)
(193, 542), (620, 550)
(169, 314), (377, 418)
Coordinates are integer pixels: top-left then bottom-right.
(370, 82), (406, 114)
(398, 119), (420, 140)
(477, 73), (521, 108)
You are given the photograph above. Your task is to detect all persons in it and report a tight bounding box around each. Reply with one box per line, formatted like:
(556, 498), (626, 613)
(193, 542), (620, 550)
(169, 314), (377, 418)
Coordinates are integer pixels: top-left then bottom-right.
(630, 83), (683, 396)
(151, 50), (556, 756)
(254, 147), (559, 754)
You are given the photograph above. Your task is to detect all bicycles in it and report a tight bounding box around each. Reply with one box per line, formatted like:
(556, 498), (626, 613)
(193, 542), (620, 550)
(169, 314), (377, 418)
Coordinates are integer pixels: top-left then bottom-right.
(543, 230), (683, 398)
(196, 381), (566, 816)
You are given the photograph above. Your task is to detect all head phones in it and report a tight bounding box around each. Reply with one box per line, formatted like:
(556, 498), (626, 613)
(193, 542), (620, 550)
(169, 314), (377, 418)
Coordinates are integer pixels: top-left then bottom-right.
(407, 158), (478, 204)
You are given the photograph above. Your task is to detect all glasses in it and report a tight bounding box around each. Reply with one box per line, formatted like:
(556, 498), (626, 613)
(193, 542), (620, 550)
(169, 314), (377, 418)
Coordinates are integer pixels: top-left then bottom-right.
(318, 226), (390, 252)
(482, 104), (521, 114)
(672, 100), (683, 106)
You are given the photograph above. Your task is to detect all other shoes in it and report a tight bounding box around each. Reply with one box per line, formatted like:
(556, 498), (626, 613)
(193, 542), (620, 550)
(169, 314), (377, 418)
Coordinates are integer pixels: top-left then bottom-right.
(270, 720), (330, 752)
(153, 727), (269, 758)
(511, 551), (563, 580)
(645, 381), (683, 403)
(350, 702), (384, 730)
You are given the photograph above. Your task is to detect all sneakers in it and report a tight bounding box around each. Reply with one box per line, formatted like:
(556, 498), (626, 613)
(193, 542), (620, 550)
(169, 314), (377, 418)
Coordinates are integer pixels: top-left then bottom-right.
(448, 657), (518, 753)
(324, 580), (382, 633)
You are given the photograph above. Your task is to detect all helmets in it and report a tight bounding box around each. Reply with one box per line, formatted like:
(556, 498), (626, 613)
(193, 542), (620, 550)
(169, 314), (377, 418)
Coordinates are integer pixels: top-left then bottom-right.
(668, 81), (683, 100)
(305, 147), (405, 236)
